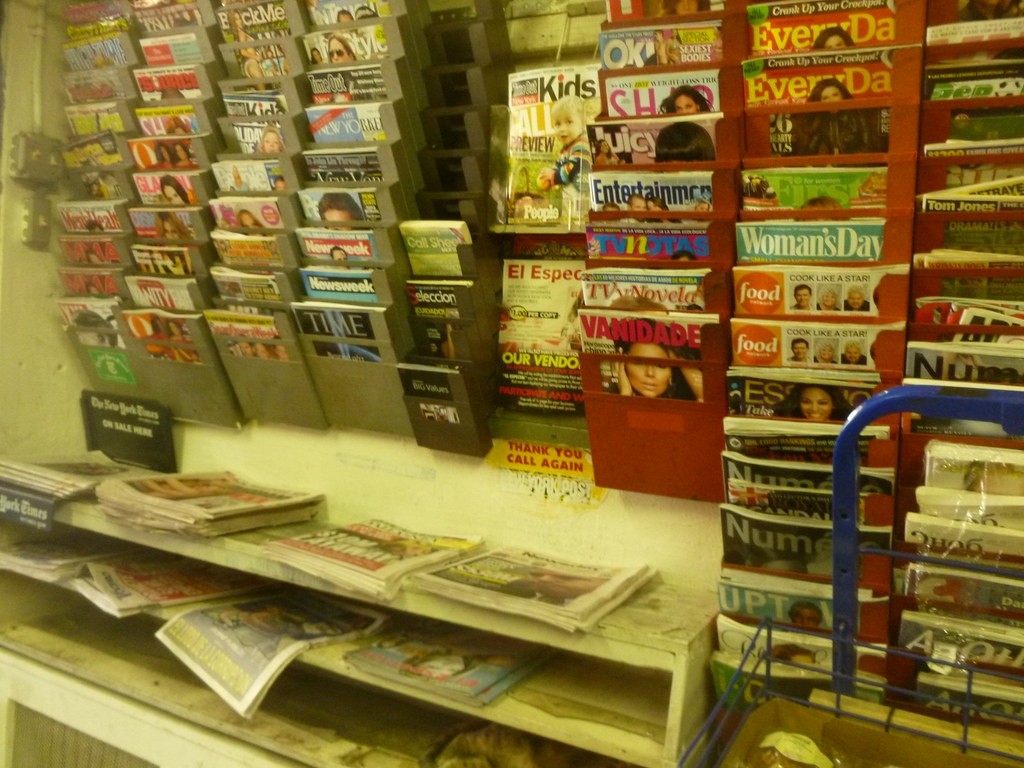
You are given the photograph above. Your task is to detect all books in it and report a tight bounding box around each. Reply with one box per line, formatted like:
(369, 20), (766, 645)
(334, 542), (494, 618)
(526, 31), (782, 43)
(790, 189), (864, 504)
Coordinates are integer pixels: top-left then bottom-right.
(0, 0), (1024, 747)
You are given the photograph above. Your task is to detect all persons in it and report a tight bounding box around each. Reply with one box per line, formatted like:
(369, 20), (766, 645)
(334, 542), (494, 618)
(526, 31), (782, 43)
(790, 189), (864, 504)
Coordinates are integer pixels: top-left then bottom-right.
(330, 246), (347, 261)
(328, 34), (356, 63)
(537, 96), (591, 195)
(655, 122), (715, 161)
(155, 212), (190, 239)
(87, 282), (100, 294)
(842, 339), (867, 364)
(660, 86), (711, 112)
(607, 318), (704, 400)
(626, 195), (647, 224)
(788, 338), (811, 362)
(260, 126), (281, 154)
(75, 309), (116, 346)
(318, 193), (364, 230)
(788, 601), (822, 627)
(810, 78), (852, 101)
(88, 249), (104, 263)
(168, 320), (187, 339)
(238, 210), (262, 228)
(311, 48), (324, 63)
(815, 27), (853, 47)
(814, 340), (838, 362)
(603, 203), (620, 223)
(272, 336), (287, 359)
(790, 285), (814, 310)
(159, 143), (171, 161)
(87, 220), (103, 232)
(596, 140), (624, 165)
(229, 166), (249, 191)
(254, 343), (274, 359)
(238, 334), (254, 356)
(772, 383), (853, 419)
(173, 143), (191, 165)
(816, 285), (841, 311)
(843, 285), (871, 311)
(647, 198), (677, 223)
(228, 8), (265, 90)
(774, 644), (814, 664)
(160, 176), (191, 206)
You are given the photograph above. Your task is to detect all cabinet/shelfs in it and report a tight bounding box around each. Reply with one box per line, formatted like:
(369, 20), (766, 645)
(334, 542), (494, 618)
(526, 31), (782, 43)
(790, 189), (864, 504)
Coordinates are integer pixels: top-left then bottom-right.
(1, 494), (716, 768)
